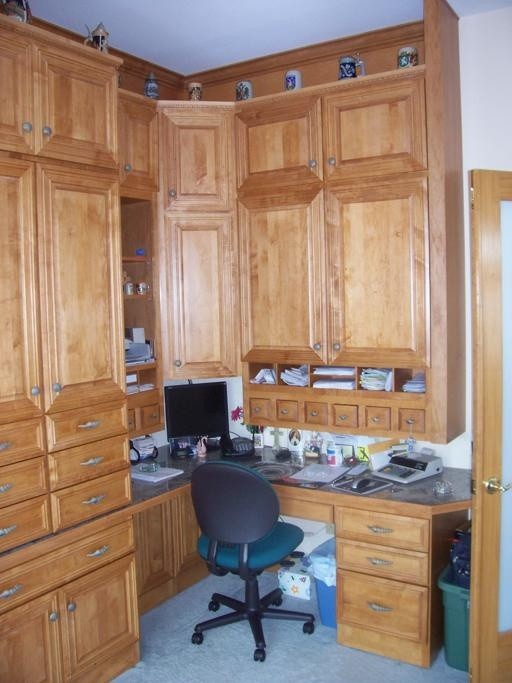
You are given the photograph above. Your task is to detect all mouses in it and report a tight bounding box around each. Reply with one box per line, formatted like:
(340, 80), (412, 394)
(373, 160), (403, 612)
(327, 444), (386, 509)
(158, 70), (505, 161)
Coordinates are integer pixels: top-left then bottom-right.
(352, 478), (368, 488)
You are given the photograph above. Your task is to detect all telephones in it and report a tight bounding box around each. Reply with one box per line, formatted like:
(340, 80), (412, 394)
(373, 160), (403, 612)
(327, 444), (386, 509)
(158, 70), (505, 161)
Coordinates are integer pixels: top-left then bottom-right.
(221, 437), (255, 457)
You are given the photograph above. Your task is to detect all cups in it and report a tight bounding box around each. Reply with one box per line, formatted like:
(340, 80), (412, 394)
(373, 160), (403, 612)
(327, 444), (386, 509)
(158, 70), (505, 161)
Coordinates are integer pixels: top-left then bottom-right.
(124, 281), (149, 296)
(2, 1), (27, 21)
(187, 82), (202, 101)
(396, 46), (418, 70)
(337, 56), (367, 78)
(234, 79), (253, 102)
(284, 69), (304, 91)
(319, 446), (342, 467)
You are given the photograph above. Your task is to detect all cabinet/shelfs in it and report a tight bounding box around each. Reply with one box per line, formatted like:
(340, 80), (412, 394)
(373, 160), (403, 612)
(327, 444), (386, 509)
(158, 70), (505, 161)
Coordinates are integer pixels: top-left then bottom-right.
(238, 64), (467, 445)
(2, 151), (127, 415)
(2, 401), (145, 682)
(116, 86), (160, 198)
(113, 192), (170, 440)
(156, 100), (241, 378)
(0, 14), (123, 166)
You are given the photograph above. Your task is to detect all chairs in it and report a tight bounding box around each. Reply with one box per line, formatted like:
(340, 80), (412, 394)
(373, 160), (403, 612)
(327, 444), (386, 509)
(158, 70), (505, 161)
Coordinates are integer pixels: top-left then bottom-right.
(187, 460), (318, 664)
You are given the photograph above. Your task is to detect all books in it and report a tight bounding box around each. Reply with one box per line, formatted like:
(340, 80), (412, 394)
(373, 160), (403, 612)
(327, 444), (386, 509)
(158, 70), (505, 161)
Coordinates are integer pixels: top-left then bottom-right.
(251, 364), (426, 393)
(291, 463), (351, 484)
(125, 343), (156, 394)
(130, 462), (184, 483)
(331, 474), (394, 495)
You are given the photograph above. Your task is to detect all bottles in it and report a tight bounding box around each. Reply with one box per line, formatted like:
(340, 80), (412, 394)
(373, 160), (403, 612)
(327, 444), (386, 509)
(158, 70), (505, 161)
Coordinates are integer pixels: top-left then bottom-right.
(144, 73), (160, 99)
(304, 440), (322, 459)
(92, 23), (110, 54)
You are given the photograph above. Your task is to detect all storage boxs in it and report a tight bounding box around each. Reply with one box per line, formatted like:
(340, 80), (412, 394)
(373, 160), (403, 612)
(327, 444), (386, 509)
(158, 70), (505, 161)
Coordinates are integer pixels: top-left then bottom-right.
(274, 555), (317, 601)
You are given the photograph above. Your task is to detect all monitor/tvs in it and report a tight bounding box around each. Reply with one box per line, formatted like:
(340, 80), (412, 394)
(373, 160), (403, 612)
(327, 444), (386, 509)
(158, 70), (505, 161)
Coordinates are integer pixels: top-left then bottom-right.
(165, 381), (229, 443)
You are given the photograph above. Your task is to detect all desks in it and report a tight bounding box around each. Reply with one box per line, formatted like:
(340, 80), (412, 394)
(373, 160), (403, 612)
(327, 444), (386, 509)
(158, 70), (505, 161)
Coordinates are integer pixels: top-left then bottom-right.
(129, 442), (474, 670)
